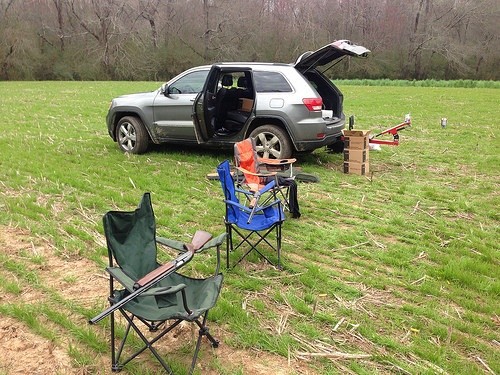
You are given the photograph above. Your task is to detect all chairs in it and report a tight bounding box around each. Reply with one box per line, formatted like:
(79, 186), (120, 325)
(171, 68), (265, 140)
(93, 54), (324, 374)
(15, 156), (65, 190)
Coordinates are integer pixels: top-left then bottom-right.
(214, 74), (252, 131)
(102, 192), (225, 375)
(232, 136), (297, 212)
(217, 157), (287, 268)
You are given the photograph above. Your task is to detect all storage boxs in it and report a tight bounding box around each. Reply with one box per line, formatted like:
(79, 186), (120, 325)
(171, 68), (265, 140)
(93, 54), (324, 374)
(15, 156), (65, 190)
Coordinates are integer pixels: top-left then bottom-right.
(343, 128), (372, 175)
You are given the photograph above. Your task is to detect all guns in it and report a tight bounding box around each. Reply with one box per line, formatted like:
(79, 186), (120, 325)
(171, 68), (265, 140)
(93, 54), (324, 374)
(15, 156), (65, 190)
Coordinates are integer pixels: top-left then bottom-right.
(246, 190), (260, 224)
(88, 229), (212, 324)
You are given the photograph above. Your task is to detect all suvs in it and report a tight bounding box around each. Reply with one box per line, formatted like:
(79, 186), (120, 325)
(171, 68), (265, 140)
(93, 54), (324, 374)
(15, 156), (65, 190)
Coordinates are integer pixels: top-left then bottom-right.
(105, 35), (372, 160)
